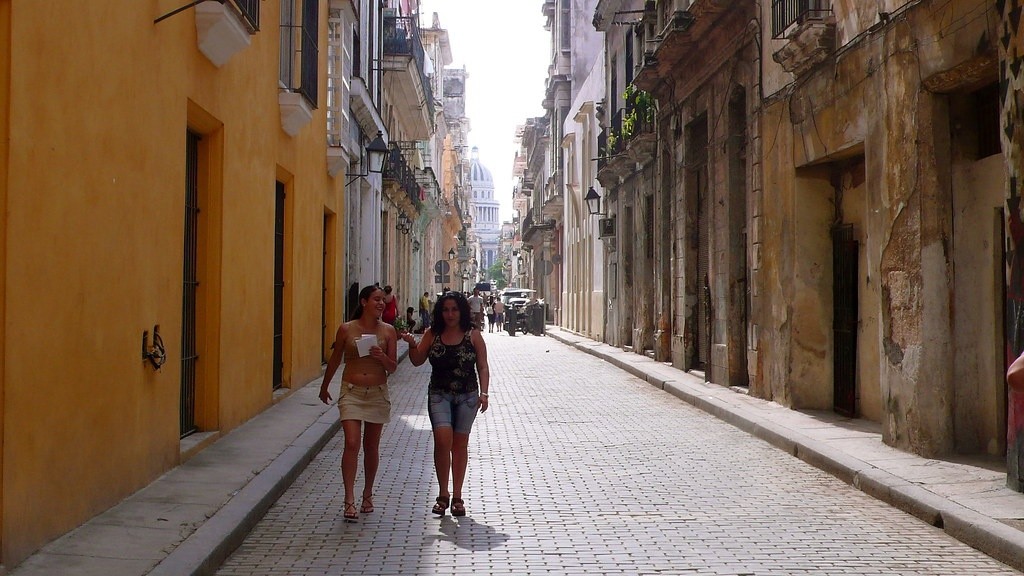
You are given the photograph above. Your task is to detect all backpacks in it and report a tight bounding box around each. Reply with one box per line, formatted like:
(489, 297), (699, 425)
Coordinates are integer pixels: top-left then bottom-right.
(486, 302), (495, 314)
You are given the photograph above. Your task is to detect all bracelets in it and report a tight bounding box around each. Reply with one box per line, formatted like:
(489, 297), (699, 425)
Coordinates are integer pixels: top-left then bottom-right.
(409, 345), (417, 348)
(380, 354), (387, 361)
(480, 393), (488, 396)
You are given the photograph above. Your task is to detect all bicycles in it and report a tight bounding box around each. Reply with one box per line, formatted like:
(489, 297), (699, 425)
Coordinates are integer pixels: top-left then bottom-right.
(515, 317), (529, 334)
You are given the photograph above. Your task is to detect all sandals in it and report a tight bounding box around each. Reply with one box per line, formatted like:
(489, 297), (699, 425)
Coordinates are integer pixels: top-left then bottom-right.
(359, 493), (374, 513)
(432, 496), (449, 515)
(450, 497), (466, 515)
(344, 500), (358, 521)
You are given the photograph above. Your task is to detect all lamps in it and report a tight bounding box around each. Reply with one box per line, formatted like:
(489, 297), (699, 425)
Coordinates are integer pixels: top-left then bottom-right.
(448, 247), (456, 260)
(395, 209), (412, 235)
(584, 185), (607, 216)
(518, 254), (524, 264)
(345, 131), (391, 177)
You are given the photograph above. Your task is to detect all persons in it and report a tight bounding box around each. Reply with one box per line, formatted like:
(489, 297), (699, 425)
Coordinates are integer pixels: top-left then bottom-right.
(381, 286), (399, 325)
(485, 296), (496, 333)
(464, 287), (488, 331)
(419, 292), (430, 328)
(407, 307), (416, 333)
(494, 297), (505, 332)
(400, 291), (489, 516)
(318, 285), (398, 519)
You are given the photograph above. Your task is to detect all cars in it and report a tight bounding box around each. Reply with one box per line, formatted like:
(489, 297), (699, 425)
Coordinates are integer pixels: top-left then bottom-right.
(504, 298), (534, 331)
